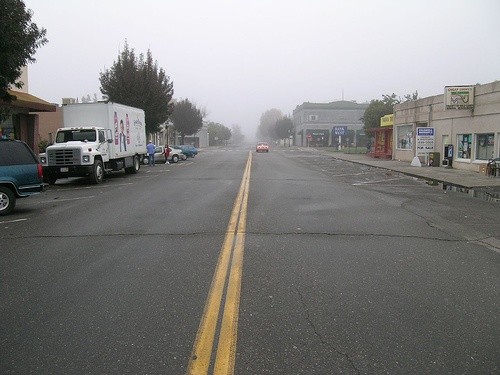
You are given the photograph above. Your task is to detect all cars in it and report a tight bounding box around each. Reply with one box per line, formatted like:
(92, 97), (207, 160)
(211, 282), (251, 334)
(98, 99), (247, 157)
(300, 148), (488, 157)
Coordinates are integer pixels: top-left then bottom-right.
(256, 142), (269, 153)
(142, 144), (198, 166)
(0, 138), (49, 216)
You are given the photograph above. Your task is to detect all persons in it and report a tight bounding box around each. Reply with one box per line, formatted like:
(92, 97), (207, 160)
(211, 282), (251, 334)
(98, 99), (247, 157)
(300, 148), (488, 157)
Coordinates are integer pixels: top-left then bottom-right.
(147, 140), (156, 166)
(161, 144), (172, 165)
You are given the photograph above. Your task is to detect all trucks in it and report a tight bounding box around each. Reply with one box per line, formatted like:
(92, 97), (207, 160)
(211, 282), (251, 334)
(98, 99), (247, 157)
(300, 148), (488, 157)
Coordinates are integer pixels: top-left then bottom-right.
(38, 101), (148, 185)
